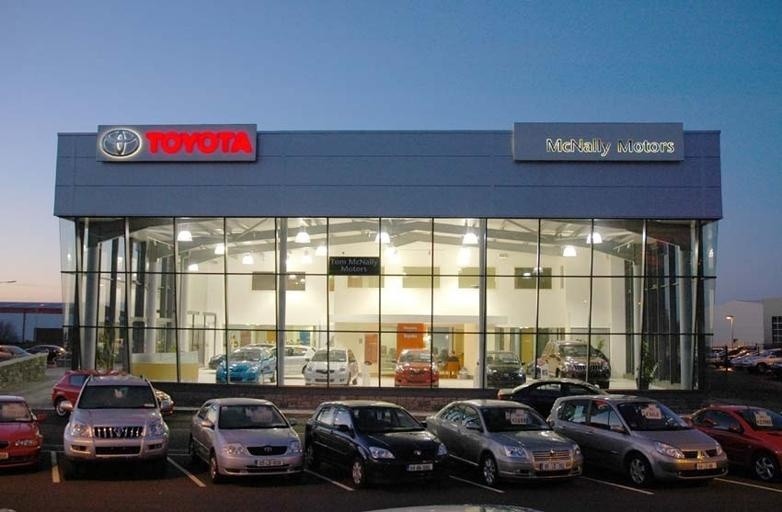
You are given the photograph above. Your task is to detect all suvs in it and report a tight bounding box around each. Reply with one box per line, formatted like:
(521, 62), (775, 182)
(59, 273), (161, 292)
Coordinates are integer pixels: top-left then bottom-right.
(62, 373), (169, 481)
(742, 348), (782, 373)
(541, 340), (611, 388)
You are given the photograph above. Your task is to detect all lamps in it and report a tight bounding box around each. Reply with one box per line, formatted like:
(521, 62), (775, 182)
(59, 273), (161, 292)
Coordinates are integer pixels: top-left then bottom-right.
(562, 244), (577, 259)
(532, 266), (543, 274)
(374, 231), (391, 244)
(214, 243), (228, 255)
(178, 230), (193, 242)
(461, 232), (478, 246)
(585, 232), (603, 246)
(294, 232), (311, 244)
(241, 253), (254, 265)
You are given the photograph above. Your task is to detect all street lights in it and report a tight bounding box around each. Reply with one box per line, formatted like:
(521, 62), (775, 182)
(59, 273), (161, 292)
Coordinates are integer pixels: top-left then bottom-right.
(726, 314), (736, 349)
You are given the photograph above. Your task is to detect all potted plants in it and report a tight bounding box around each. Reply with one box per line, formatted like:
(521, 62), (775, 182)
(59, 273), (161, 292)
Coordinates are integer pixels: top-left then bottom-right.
(635, 339), (657, 389)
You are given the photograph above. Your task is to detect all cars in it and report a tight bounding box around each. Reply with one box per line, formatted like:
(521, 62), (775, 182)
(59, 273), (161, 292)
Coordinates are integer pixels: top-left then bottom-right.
(727, 349), (755, 361)
(546, 395), (729, 486)
(476, 350), (526, 388)
(303, 400), (448, 488)
(742, 352), (766, 367)
(208, 344), (274, 370)
(683, 404), (782, 483)
(714, 349), (741, 368)
(215, 347), (277, 385)
(304, 346), (359, 386)
(270, 345), (316, 375)
(51, 370), (174, 419)
(497, 379), (610, 419)
(26, 345), (65, 364)
(0, 345), (30, 361)
(425, 400), (583, 485)
(0, 395), (48, 469)
(391, 348), (442, 388)
(731, 352), (758, 368)
(188, 398), (302, 485)
